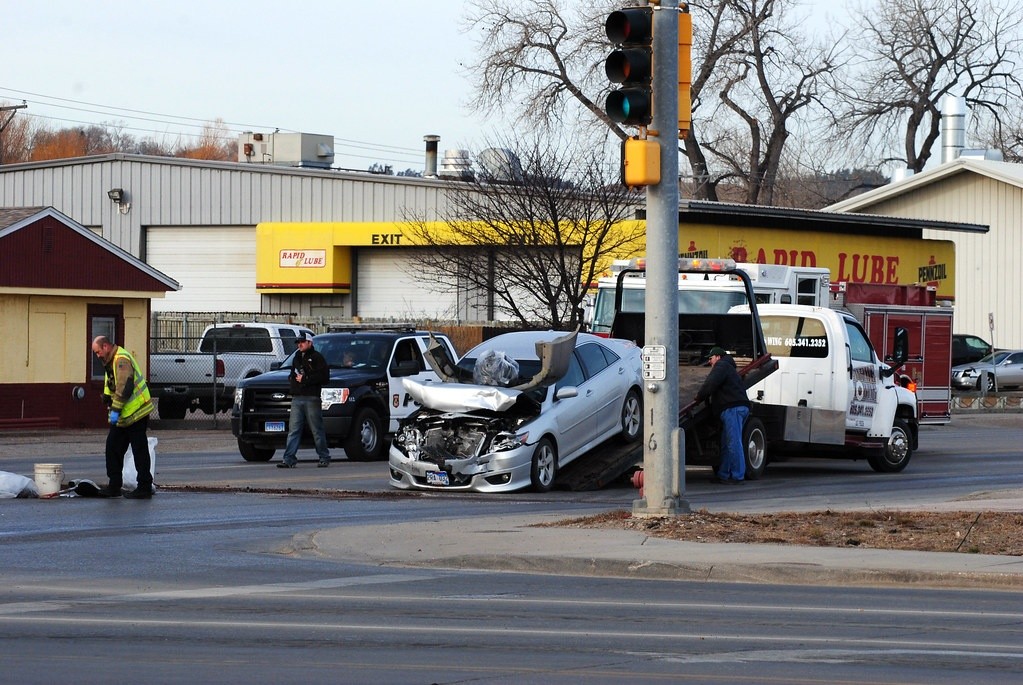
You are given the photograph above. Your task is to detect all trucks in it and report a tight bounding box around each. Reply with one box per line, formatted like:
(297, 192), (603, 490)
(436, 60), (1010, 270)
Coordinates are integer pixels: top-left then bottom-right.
(551, 269), (919, 495)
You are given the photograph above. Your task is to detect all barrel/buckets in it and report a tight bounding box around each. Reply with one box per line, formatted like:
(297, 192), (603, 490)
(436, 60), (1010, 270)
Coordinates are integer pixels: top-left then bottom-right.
(33, 463), (63, 499)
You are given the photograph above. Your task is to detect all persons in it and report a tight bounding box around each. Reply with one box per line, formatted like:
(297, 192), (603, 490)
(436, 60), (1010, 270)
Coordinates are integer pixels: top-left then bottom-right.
(694, 347), (750, 486)
(92, 335), (154, 500)
(276, 332), (331, 468)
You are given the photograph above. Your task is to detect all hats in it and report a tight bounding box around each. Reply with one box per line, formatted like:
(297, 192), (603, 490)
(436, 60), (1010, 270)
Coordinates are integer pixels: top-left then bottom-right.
(295, 333), (314, 343)
(704, 347), (726, 358)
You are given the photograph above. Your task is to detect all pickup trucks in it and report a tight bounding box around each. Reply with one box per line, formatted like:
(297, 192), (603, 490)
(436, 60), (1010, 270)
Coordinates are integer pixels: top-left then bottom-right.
(151, 323), (317, 420)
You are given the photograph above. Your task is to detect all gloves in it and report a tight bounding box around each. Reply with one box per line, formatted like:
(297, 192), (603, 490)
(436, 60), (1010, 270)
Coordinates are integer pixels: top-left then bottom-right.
(110, 410), (119, 425)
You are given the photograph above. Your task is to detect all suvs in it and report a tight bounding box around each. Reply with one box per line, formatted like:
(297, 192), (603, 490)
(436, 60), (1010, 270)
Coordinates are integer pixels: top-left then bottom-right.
(230, 323), (460, 462)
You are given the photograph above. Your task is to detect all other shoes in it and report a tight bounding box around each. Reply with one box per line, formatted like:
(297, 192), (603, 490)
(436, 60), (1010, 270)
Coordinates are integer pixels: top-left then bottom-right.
(318, 458), (329, 467)
(722, 478), (745, 485)
(709, 477), (722, 484)
(277, 462), (295, 468)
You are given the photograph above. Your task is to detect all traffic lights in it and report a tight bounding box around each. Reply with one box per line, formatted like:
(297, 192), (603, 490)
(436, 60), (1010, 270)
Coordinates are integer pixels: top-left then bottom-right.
(620, 137), (661, 188)
(603, 6), (652, 126)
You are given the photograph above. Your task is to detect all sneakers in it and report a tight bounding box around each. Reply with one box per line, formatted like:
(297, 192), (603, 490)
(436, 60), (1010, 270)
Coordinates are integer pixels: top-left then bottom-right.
(98, 487), (121, 497)
(124, 485), (152, 499)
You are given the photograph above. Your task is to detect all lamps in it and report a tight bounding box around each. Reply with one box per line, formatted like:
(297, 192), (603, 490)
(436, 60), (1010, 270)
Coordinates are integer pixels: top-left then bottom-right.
(108, 189), (122, 199)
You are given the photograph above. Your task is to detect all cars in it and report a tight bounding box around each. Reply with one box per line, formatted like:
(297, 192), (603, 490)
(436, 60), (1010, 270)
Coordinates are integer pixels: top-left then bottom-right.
(389, 331), (645, 495)
(952, 333), (1023, 391)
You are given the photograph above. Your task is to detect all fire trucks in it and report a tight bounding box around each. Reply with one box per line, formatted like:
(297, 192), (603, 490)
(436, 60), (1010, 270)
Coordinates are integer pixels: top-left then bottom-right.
(584, 256), (955, 427)
